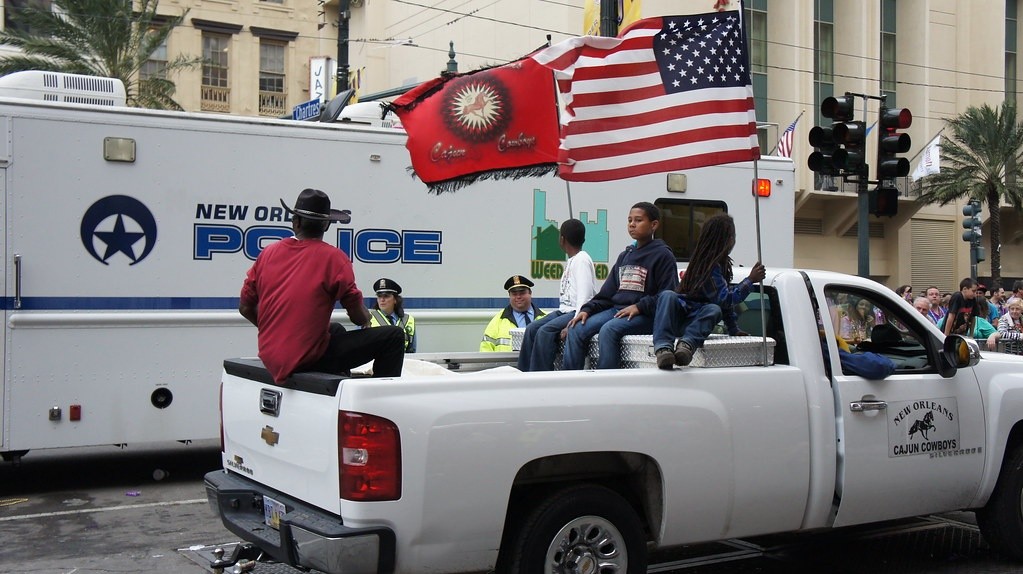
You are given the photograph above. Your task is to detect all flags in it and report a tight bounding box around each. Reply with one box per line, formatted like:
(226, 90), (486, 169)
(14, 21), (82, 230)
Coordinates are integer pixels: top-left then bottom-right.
(383, 41), (560, 194)
(777, 120), (796, 158)
(912, 134), (940, 182)
(527, 10), (761, 183)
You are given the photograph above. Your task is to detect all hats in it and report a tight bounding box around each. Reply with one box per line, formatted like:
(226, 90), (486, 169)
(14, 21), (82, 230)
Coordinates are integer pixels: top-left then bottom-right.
(280, 189), (350, 221)
(978, 285), (987, 293)
(504, 275), (534, 292)
(373, 278), (402, 295)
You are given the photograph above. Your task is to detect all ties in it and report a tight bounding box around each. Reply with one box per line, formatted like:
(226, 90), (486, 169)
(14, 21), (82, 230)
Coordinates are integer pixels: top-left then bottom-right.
(522, 311), (531, 325)
(386, 316), (394, 325)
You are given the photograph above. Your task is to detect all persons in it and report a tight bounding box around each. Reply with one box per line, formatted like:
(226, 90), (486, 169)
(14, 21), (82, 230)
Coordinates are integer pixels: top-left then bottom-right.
(356, 278), (417, 354)
(654, 215), (766, 369)
(562, 202), (679, 371)
(239, 189), (405, 384)
(517, 218), (598, 371)
(479, 275), (548, 352)
(818, 278), (1023, 355)
(820, 329), (894, 381)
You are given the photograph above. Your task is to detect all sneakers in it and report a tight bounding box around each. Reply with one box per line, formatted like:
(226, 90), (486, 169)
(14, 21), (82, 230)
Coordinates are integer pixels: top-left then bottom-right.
(656, 348), (675, 368)
(674, 342), (693, 366)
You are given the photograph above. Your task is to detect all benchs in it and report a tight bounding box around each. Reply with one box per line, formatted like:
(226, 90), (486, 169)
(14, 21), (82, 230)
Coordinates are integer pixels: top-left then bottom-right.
(510, 328), (776, 371)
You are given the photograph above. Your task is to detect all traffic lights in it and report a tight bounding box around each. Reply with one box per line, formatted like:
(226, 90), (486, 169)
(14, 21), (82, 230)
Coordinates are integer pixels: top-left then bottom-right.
(962, 201), (981, 242)
(876, 106), (914, 183)
(807, 95), (866, 178)
(869, 184), (900, 218)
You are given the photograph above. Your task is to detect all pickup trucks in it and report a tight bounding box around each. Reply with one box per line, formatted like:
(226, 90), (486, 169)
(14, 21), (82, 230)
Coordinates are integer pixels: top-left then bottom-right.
(201, 269), (1023, 573)
